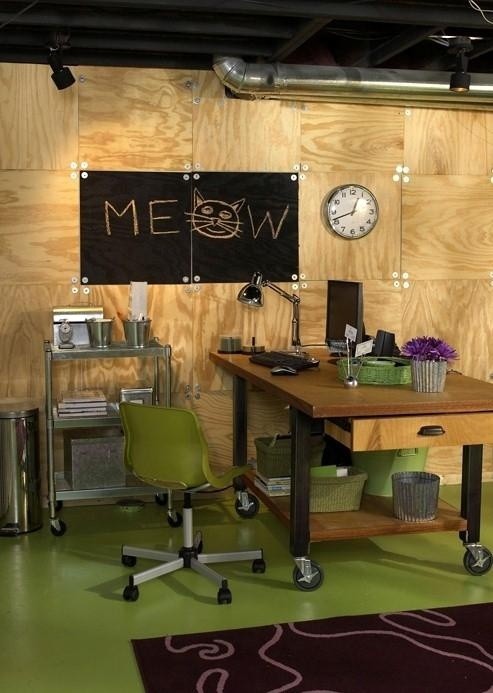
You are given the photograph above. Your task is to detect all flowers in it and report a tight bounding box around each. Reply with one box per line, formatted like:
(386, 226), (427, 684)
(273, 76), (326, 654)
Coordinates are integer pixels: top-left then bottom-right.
(401, 335), (460, 364)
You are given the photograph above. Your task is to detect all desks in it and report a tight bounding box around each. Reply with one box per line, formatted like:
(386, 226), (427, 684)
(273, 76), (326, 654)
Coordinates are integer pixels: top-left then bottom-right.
(207, 343), (493, 592)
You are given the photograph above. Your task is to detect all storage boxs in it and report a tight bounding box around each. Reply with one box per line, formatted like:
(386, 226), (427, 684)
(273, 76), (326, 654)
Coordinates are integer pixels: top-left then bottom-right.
(305, 465), (371, 516)
(62, 427), (127, 491)
(252, 432), (328, 484)
(52, 304), (104, 349)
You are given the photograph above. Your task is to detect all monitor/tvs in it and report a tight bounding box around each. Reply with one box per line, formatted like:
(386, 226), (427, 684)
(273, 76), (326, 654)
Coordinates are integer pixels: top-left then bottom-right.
(325, 279), (364, 356)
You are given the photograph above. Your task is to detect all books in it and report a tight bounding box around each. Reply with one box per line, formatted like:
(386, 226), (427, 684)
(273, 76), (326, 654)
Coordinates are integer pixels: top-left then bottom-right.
(54, 388), (108, 418)
(253, 471), (292, 496)
(310, 465), (348, 478)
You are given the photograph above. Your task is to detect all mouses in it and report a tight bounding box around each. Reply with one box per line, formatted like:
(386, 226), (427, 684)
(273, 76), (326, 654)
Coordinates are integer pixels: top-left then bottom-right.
(270, 366), (298, 376)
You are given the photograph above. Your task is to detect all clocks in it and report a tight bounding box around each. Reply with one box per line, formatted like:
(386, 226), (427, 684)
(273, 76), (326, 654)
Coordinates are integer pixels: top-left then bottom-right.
(323, 182), (381, 241)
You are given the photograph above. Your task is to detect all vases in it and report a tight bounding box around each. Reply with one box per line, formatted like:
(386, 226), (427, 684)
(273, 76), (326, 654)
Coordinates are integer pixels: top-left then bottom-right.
(410, 357), (449, 395)
(390, 469), (442, 526)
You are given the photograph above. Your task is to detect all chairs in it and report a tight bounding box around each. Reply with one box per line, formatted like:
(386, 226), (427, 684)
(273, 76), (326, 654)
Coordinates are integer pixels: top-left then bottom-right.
(113, 399), (267, 605)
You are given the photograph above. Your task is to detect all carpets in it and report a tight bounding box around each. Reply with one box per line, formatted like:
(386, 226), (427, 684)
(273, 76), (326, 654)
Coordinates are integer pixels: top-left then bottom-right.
(130, 602), (492, 693)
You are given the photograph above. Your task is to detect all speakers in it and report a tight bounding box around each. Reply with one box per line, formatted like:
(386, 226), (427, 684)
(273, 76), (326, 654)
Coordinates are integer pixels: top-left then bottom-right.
(374, 329), (395, 357)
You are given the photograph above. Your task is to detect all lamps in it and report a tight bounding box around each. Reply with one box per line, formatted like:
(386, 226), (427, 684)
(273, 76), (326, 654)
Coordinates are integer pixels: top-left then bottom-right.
(46, 56), (76, 91)
(235, 271), (301, 346)
(448, 53), (472, 94)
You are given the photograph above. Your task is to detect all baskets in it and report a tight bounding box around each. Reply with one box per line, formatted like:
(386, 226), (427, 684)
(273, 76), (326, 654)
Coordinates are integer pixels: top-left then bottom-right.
(254, 434), (326, 478)
(308, 466), (368, 513)
(336, 355), (412, 384)
(410, 359), (447, 393)
(393, 471), (440, 522)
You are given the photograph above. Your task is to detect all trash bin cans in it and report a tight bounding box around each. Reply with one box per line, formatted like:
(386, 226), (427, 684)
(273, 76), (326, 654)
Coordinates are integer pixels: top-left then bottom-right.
(0, 402), (42, 536)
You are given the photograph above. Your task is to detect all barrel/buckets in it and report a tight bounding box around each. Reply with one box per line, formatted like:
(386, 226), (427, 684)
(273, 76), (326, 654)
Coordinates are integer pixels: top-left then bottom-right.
(124, 319), (152, 349)
(85, 318), (114, 348)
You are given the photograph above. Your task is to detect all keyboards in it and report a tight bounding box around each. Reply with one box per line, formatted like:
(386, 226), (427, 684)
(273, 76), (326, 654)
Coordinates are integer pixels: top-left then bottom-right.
(248, 351), (319, 370)
